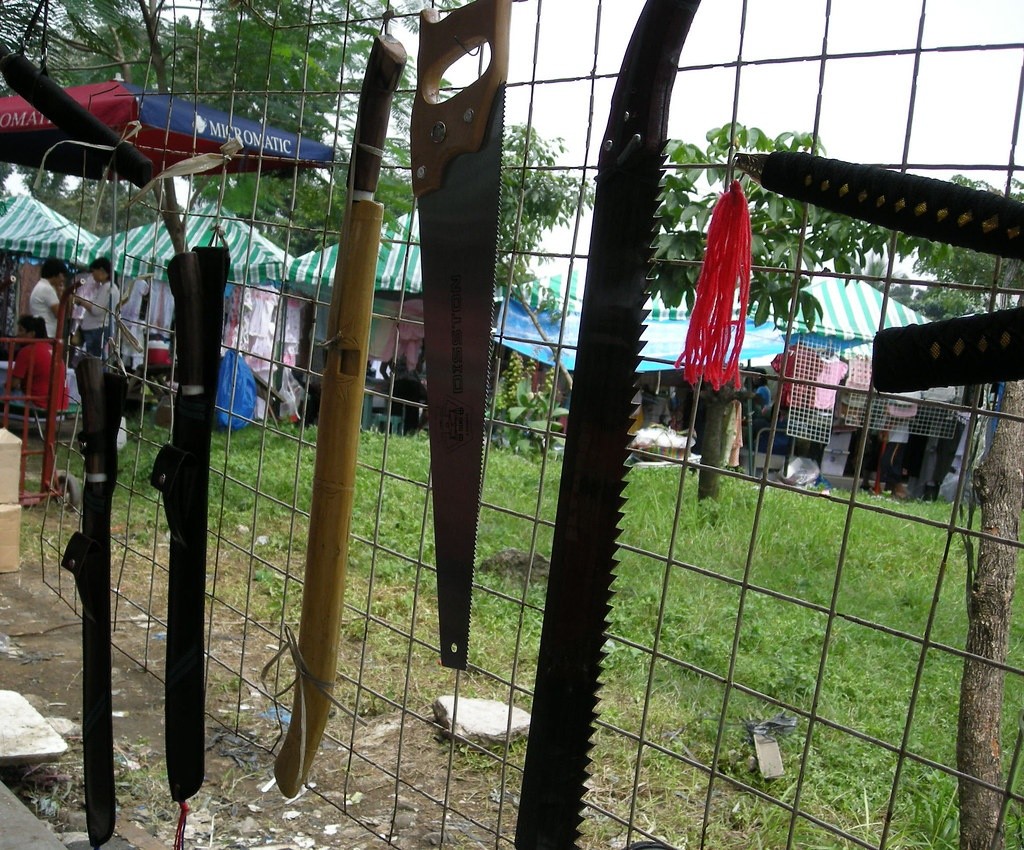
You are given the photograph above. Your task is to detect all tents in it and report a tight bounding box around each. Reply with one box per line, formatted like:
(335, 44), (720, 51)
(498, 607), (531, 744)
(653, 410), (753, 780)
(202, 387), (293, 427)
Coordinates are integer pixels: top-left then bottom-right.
(0, 195), (933, 478)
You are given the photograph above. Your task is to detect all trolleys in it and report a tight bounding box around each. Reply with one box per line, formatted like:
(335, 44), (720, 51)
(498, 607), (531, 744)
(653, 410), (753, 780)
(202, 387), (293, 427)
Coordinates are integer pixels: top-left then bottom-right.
(0, 335), (82, 513)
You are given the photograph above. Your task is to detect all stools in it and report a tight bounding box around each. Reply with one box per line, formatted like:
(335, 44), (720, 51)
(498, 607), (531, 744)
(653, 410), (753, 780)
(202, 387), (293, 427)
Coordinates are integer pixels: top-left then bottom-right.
(373, 413), (405, 438)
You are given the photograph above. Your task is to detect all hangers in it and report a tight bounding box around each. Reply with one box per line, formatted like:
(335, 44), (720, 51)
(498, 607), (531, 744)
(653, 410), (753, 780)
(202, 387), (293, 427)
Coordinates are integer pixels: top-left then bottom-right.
(775, 331), (847, 371)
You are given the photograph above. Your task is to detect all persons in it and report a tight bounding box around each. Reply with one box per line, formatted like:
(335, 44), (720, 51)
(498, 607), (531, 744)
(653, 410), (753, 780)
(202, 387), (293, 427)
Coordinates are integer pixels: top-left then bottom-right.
(29, 257), (70, 346)
(5, 314), (69, 411)
(869, 420), (910, 498)
(380, 354), (420, 436)
(74, 256), (120, 357)
(148, 333), (172, 365)
(755, 378), (771, 409)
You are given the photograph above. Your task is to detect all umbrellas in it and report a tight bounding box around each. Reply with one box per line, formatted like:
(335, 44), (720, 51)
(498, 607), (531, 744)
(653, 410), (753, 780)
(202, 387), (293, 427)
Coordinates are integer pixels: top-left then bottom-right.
(0, 72), (336, 373)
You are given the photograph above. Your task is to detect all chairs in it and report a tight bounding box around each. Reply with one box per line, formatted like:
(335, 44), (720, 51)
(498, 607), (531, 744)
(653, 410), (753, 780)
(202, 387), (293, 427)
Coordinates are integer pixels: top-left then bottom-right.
(0, 395), (83, 450)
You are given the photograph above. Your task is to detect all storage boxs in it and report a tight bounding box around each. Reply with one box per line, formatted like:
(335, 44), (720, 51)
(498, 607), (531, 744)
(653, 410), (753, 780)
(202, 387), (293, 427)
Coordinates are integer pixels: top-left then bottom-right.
(811, 431), (853, 477)
